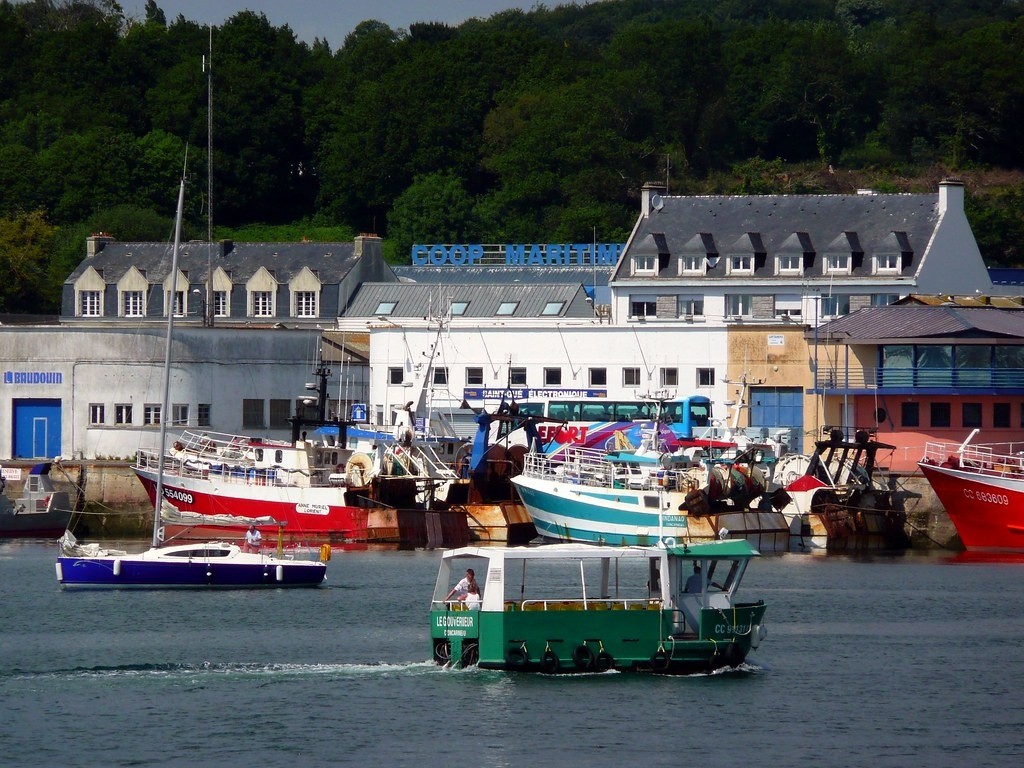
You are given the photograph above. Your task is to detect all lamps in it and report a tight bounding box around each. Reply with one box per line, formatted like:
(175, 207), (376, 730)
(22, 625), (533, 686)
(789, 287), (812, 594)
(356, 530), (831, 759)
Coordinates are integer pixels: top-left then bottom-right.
(782, 314), (801, 326)
(275, 323), (288, 329)
(585, 298), (603, 316)
(377, 316), (401, 327)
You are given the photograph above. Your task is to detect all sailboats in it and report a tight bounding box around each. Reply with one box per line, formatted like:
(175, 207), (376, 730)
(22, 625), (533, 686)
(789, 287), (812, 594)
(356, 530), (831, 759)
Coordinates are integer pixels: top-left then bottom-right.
(54, 180), (330, 589)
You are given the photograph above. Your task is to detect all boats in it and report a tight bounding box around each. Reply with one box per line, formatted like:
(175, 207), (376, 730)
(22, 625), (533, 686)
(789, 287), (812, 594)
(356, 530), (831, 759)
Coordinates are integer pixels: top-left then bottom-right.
(129, 283), (919, 558)
(916, 428), (1024, 553)
(0, 451), (87, 536)
(429, 527), (769, 672)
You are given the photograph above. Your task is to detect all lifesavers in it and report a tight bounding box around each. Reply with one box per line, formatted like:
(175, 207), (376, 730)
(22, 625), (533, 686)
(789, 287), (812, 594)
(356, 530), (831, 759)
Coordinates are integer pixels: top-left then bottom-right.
(725, 643), (744, 669)
(540, 651), (559, 674)
(595, 653), (614, 673)
(709, 653), (725, 669)
(650, 651), (671, 674)
(572, 645), (594, 670)
(505, 647), (529, 669)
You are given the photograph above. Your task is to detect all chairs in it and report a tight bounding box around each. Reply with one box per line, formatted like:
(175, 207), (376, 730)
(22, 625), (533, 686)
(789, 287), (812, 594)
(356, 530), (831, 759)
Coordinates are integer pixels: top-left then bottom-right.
(452, 600), (659, 611)
(533, 410), (706, 422)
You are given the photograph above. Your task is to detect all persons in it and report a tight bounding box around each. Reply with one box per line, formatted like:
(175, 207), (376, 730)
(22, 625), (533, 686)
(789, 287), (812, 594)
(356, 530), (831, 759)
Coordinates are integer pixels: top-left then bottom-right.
(442, 568), (481, 605)
(684, 566), (723, 593)
(200, 433), (248, 451)
(457, 583), (480, 610)
(243, 524), (262, 554)
(647, 568), (660, 591)
